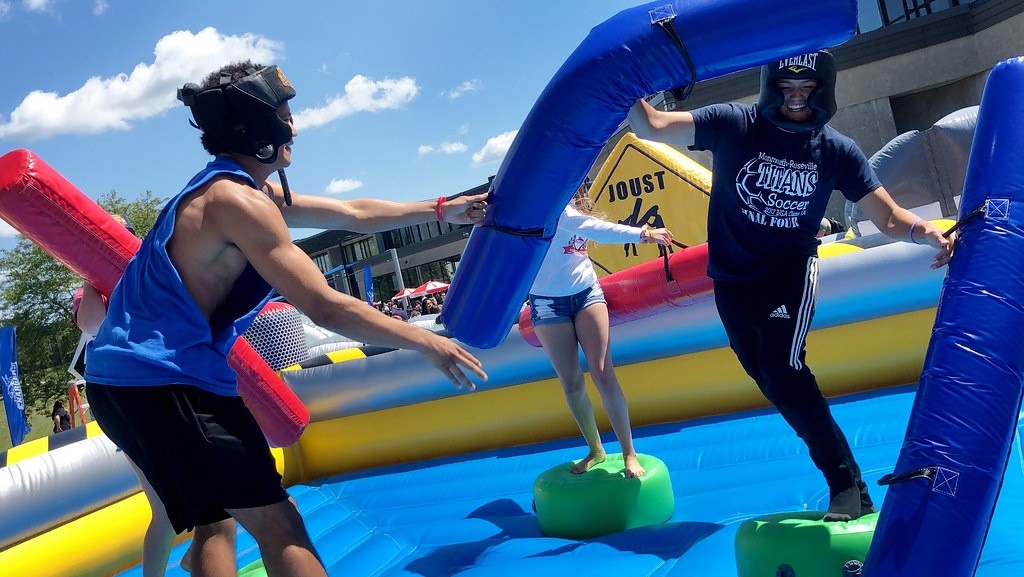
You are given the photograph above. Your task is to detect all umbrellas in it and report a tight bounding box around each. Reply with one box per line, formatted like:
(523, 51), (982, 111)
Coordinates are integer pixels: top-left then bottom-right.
(409, 280), (451, 298)
(391, 288), (416, 301)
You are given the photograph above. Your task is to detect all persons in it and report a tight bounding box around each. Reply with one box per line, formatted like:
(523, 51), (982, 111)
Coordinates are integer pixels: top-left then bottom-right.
(72, 214), (196, 576)
(528, 204), (672, 478)
(51, 399), (71, 435)
(83, 62), (492, 577)
(630, 50), (960, 519)
(816, 217), (831, 237)
(378, 293), (447, 323)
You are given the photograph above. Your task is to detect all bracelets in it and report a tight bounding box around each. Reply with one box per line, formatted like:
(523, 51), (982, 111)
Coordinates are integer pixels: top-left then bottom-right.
(641, 226), (647, 244)
(648, 230), (655, 243)
(437, 196), (446, 221)
(909, 218), (924, 244)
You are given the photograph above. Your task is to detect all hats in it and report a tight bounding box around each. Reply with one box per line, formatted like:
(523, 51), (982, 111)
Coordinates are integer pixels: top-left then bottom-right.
(109, 214), (136, 235)
(756, 48), (838, 134)
